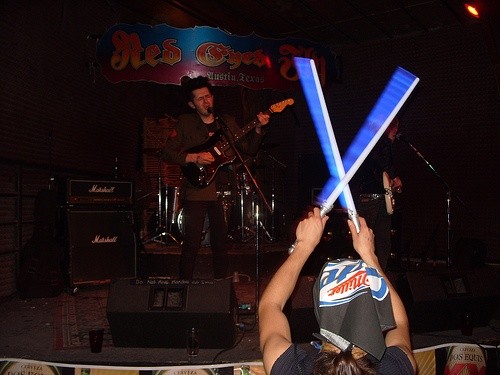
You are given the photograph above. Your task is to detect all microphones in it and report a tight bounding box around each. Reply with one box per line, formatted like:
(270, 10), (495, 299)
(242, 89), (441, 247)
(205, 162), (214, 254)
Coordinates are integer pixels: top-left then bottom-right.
(395, 133), (412, 143)
(207, 107), (217, 114)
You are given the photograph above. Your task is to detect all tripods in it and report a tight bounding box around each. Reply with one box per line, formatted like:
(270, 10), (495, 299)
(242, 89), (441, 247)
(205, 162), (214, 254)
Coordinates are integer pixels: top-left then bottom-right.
(225, 183), (274, 248)
(142, 172), (184, 249)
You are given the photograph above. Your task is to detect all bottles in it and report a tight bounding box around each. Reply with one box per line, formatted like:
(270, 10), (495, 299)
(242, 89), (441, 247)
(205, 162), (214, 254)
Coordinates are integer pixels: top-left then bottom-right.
(48, 177), (57, 192)
(113, 155), (120, 181)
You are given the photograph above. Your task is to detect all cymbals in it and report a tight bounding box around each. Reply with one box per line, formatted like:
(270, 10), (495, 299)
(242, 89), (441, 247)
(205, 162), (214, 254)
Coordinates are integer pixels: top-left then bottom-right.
(244, 142), (282, 152)
(142, 146), (163, 157)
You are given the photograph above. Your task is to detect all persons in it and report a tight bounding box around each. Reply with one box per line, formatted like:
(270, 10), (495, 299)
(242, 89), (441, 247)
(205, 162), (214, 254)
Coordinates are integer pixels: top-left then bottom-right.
(258, 208), (417, 375)
(168, 77), (271, 282)
(358, 116), (409, 275)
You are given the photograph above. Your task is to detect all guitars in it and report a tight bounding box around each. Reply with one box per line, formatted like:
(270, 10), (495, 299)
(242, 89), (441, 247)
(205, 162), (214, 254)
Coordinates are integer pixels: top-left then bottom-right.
(382, 170), (396, 214)
(181, 97), (295, 189)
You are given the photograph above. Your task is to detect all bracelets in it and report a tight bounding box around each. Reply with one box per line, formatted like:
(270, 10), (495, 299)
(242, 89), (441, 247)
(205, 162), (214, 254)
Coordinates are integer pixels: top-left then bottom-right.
(196, 155), (201, 164)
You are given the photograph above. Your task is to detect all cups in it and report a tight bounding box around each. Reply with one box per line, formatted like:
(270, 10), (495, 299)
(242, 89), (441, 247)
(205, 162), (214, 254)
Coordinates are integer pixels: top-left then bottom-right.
(186, 330), (200, 358)
(89, 327), (104, 353)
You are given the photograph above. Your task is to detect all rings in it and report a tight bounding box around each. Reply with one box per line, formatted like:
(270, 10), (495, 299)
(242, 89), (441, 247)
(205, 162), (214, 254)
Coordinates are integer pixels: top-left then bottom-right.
(397, 188), (399, 190)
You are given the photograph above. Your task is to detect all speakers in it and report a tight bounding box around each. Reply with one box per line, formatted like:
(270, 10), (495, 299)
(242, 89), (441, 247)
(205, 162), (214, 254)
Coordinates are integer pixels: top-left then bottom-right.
(395, 268), (500, 334)
(105, 283), (238, 350)
(283, 273), (320, 343)
(54, 207), (138, 289)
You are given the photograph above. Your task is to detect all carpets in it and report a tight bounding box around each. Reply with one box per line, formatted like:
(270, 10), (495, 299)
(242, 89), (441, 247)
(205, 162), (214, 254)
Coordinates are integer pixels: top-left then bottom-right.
(54, 288), (114, 351)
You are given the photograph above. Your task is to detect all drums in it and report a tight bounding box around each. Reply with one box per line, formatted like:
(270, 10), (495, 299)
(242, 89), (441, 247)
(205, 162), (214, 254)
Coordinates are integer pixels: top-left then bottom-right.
(161, 185), (182, 213)
(176, 191), (230, 246)
(229, 170), (261, 196)
